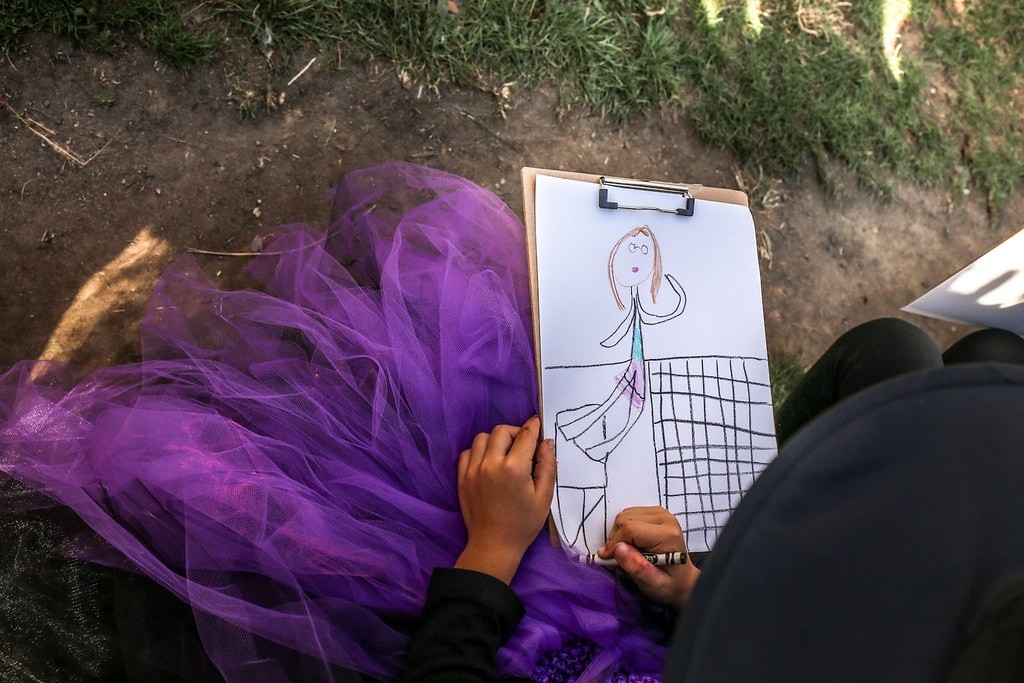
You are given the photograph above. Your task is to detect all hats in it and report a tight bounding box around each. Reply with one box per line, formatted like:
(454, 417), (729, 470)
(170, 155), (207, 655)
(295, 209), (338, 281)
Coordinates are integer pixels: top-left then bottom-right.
(664, 364), (1024, 683)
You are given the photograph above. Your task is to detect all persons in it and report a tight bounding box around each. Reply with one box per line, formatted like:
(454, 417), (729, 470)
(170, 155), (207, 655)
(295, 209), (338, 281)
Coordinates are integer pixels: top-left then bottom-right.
(397, 316), (1024, 683)
